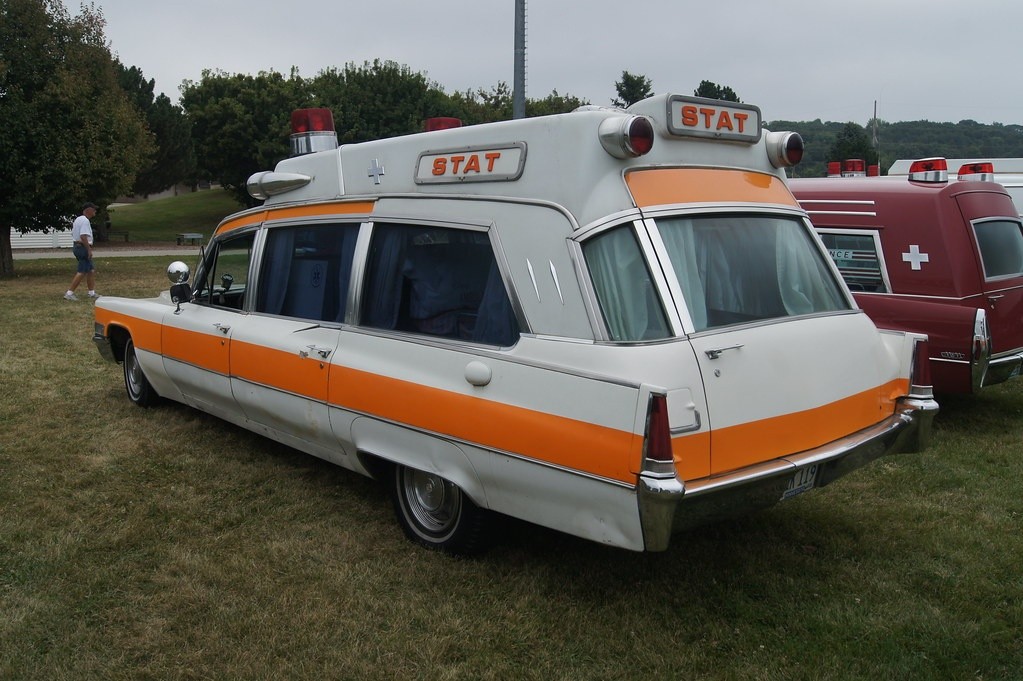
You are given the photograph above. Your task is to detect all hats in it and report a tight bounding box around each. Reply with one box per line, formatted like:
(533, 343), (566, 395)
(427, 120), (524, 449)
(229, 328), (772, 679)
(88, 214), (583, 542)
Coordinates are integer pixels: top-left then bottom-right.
(82, 202), (99, 209)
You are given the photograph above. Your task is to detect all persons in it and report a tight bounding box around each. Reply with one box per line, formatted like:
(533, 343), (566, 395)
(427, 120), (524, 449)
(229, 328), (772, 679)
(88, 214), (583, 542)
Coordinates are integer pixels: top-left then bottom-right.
(64, 202), (104, 302)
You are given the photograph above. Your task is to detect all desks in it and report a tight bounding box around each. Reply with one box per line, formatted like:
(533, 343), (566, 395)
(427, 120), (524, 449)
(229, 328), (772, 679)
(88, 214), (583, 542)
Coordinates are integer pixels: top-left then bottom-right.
(176, 233), (203, 245)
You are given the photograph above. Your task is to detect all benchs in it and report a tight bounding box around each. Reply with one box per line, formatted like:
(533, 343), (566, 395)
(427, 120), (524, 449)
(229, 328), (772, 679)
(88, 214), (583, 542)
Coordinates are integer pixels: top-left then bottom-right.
(107, 230), (129, 242)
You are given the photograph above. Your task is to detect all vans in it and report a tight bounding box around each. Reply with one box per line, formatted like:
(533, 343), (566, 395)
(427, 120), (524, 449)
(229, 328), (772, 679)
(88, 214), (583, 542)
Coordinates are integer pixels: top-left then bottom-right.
(91, 93), (941, 555)
(787, 155), (1023, 409)
(886, 158), (1023, 221)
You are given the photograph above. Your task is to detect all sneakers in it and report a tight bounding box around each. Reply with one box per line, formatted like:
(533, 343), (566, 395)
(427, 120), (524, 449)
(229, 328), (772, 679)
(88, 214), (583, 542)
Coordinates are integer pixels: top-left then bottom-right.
(87, 293), (102, 298)
(63, 292), (79, 302)
